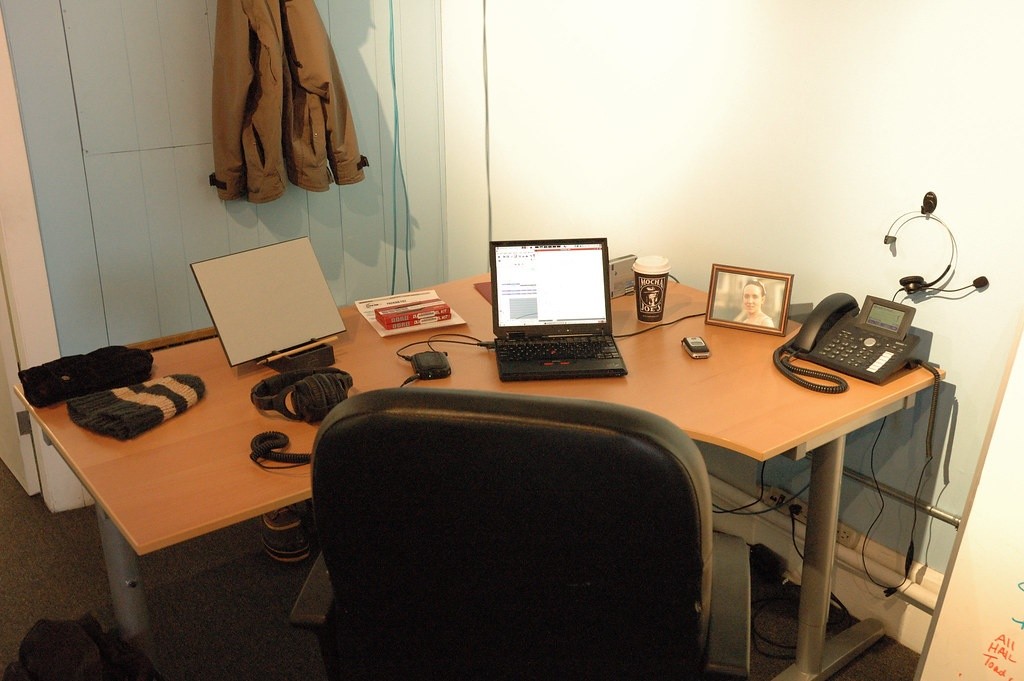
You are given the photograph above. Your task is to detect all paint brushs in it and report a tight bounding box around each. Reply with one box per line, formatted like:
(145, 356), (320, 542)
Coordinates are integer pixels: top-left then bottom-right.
(257, 337), (339, 366)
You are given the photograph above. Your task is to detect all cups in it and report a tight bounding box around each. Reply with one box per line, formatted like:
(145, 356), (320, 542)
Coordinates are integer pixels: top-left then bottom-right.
(632, 256), (671, 324)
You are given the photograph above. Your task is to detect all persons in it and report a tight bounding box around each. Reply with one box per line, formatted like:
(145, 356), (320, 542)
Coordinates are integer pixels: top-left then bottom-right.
(733, 279), (773, 329)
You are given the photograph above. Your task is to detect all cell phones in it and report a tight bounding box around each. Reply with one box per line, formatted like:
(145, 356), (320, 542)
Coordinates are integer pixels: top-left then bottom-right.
(682, 336), (710, 359)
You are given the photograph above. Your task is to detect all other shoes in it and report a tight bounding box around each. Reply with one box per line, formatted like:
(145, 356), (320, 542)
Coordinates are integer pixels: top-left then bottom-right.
(261, 503), (310, 563)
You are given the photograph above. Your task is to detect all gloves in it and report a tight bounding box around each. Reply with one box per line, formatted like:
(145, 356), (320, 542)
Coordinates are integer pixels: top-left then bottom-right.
(18, 345), (154, 408)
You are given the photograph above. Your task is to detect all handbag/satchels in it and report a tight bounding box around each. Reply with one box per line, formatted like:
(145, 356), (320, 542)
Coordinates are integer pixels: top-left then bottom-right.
(2, 612), (164, 681)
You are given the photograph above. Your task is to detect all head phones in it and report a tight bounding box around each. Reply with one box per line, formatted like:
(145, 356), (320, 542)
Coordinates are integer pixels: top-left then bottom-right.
(884, 210), (954, 294)
(251, 367), (353, 425)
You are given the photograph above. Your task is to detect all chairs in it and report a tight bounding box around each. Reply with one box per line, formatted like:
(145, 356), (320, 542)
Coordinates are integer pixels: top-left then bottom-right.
(289, 388), (751, 680)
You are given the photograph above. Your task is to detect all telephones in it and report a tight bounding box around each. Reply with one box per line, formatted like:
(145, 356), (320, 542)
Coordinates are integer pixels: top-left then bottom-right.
(793, 292), (922, 385)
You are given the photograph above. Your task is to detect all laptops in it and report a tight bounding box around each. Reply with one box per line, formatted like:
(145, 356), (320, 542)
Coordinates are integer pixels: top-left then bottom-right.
(490, 237), (628, 382)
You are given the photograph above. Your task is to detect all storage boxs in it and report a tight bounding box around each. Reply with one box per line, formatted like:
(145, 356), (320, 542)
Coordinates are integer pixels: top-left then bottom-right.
(375, 298), (452, 330)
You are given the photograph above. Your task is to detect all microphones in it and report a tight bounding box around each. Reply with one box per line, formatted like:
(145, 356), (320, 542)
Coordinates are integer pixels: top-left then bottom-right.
(921, 277), (988, 291)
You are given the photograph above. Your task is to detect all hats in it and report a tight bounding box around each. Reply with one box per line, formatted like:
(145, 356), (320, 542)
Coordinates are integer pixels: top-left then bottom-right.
(66, 372), (205, 440)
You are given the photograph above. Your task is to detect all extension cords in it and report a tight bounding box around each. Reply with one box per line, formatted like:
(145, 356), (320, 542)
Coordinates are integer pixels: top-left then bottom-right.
(765, 486), (860, 549)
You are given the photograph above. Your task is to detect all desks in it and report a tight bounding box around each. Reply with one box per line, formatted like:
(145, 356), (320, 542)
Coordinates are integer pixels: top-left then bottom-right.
(15, 270), (947, 681)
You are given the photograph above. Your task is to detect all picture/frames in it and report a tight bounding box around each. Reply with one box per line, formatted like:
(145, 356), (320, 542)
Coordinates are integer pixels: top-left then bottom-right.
(705, 264), (795, 337)
